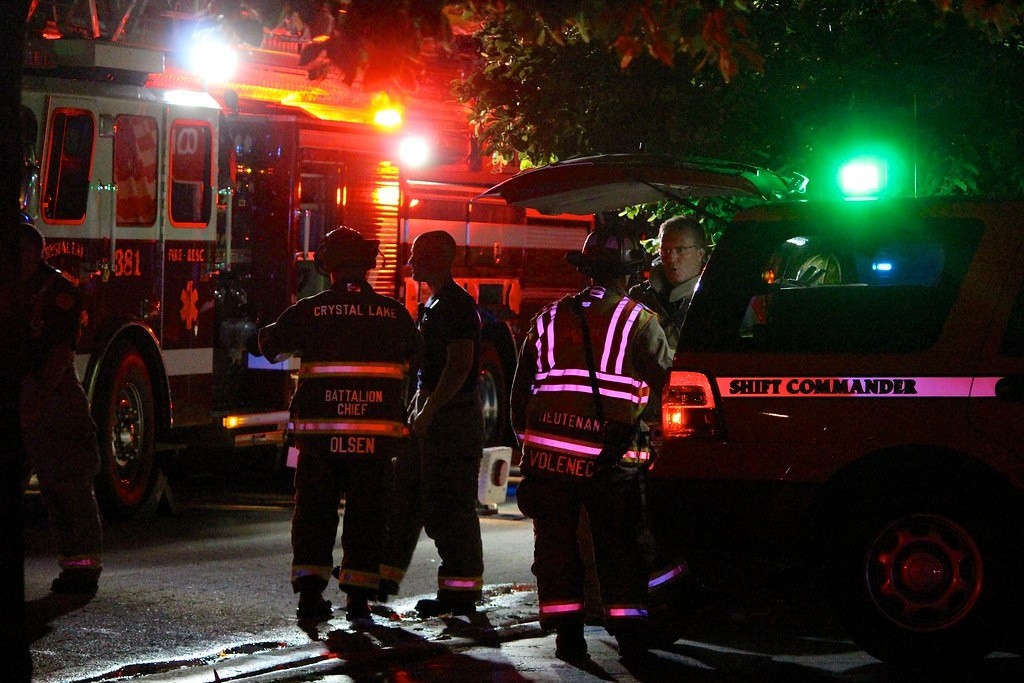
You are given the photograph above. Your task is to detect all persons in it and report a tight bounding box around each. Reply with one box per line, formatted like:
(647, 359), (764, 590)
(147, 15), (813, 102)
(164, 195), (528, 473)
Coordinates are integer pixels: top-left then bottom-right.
(331, 230), (488, 614)
(624, 214), (709, 445)
(507, 221), (677, 665)
(17, 214), (104, 605)
(232, 223), (425, 624)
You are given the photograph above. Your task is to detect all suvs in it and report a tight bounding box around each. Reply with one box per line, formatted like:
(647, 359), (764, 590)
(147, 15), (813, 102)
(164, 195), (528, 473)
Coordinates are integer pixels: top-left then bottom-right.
(470, 144), (1024, 672)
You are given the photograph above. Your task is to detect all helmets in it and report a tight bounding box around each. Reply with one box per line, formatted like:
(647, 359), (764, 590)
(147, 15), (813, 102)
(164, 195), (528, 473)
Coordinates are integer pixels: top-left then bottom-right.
(315, 227), (381, 275)
(565, 229), (645, 278)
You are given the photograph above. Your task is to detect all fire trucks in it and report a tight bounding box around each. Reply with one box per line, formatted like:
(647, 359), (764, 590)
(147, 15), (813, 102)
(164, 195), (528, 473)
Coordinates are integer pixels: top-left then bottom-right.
(0, 1), (660, 532)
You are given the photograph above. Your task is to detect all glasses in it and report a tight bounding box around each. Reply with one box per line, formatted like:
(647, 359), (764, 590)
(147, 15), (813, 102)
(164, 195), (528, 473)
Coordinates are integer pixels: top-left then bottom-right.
(659, 245), (699, 258)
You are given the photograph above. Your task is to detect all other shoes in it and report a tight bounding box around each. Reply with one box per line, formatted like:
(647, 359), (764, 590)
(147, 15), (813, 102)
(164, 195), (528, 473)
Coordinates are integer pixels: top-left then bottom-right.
(347, 591), (371, 620)
(52, 573), (98, 594)
(416, 598), (475, 615)
(614, 628), (649, 657)
(296, 588), (332, 618)
(555, 629), (588, 657)
